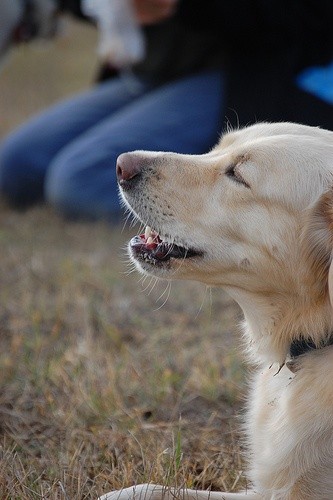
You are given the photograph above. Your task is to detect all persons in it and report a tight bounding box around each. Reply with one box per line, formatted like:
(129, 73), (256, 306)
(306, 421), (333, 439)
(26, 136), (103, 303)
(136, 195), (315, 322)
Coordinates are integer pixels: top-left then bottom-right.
(0, 1), (331, 225)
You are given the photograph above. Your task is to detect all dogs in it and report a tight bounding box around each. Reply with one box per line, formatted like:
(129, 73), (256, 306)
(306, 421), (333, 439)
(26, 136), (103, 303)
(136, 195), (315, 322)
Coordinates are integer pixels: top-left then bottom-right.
(94, 122), (333, 500)
